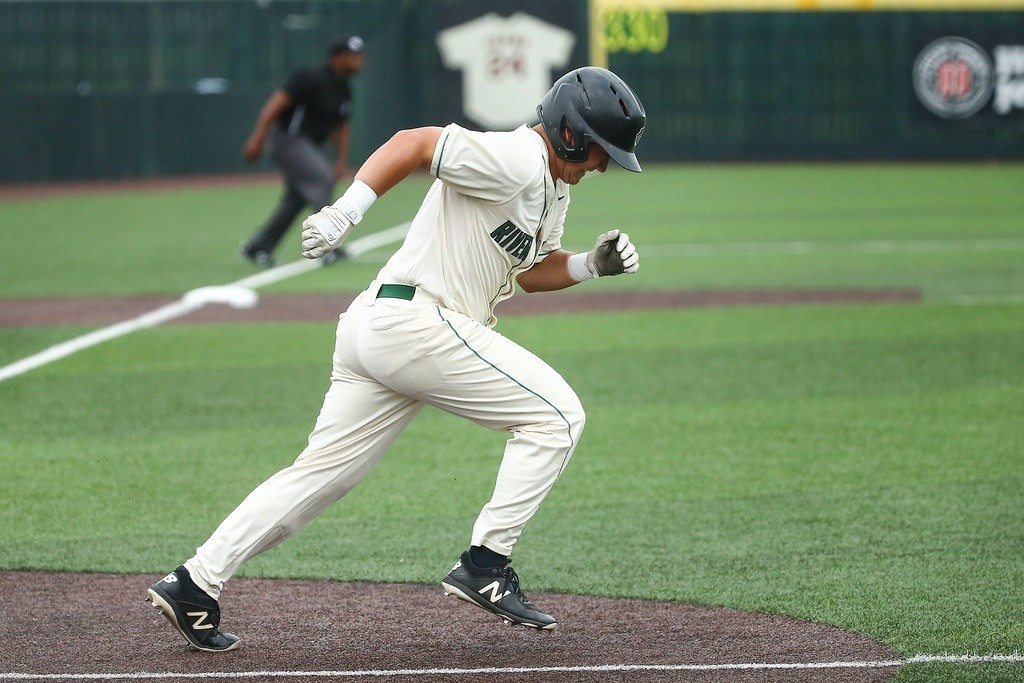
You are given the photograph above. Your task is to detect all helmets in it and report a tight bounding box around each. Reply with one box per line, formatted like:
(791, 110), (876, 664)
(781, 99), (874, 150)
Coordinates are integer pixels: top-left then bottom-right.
(536, 66), (646, 173)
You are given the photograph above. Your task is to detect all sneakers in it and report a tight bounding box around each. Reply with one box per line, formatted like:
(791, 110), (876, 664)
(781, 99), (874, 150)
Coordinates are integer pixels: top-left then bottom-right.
(442, 551), (557, 635)
(145, 566), (241, 653)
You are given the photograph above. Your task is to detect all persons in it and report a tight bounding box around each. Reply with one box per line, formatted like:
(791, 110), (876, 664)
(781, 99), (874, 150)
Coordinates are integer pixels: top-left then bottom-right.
(242, 37), (364, 268)
(147, 67), (645, 651)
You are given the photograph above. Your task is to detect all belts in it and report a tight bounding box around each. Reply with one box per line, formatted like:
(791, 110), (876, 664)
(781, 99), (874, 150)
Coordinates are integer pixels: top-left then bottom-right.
(376, 284), (416, 300)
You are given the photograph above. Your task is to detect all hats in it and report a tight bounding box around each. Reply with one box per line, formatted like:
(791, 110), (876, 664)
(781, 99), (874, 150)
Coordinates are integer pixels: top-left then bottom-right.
(327, 33), (364, 53)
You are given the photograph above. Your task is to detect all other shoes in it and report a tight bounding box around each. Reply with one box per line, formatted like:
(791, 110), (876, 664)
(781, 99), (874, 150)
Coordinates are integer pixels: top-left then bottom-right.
(323, 249), (353, 265)
(240, 243), (278, 270)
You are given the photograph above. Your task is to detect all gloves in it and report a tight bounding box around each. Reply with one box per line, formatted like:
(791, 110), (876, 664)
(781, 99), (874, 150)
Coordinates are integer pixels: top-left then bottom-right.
(301, 197), (363, 259)
(587, 229), (640, 278)
